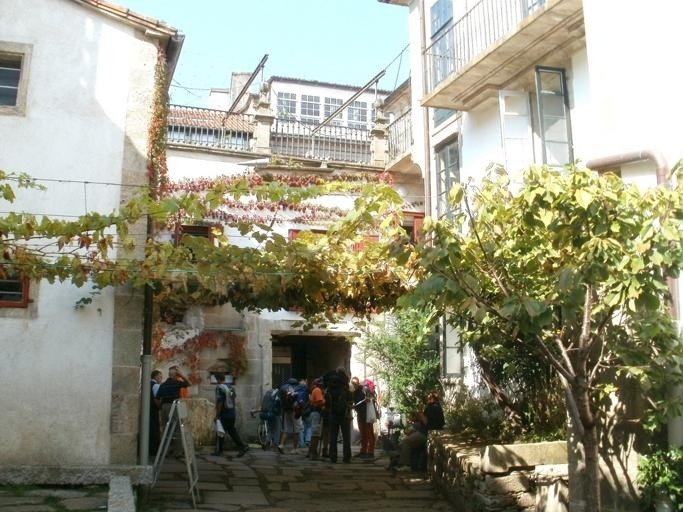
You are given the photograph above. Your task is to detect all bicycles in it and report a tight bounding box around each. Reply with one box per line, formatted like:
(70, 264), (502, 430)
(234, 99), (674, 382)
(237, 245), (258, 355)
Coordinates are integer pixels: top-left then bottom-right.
(248, 408), (270, 447)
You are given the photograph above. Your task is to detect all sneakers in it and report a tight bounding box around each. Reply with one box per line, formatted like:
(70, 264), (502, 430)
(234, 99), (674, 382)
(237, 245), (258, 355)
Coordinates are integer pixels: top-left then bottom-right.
(393, 465), (412, 472)
(236, 445), (249, 458)
(277, 444), (299, 456)
(353, 453), (374, 459)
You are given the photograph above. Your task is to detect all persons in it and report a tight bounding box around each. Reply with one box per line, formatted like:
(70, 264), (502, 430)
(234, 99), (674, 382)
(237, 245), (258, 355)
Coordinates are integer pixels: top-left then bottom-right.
(150, 371), (163, 456)
(212, 374), (251, 459)
(259, 364), (381, 463)
(393, 391), (444, 472)
(156, 366), (191, 422)
